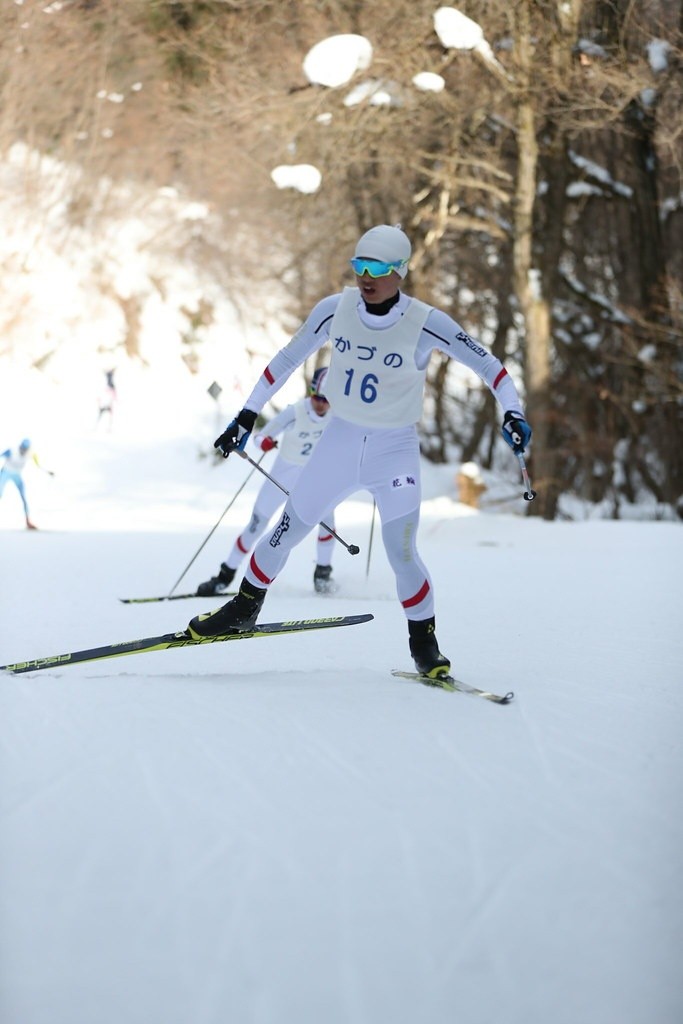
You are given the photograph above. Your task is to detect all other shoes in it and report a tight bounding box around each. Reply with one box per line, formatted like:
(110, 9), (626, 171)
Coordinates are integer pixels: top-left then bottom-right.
(27, 522), (36, 529)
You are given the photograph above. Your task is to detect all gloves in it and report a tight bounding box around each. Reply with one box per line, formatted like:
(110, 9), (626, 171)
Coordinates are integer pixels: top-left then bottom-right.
(261, 437), (276, 452)
(214, 409), (257, 458)
(501, 410), (532, 456)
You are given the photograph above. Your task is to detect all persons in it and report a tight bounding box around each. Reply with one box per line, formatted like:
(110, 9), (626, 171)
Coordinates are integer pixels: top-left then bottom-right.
(0, 438), (55, 531)
(197, 366), (345, 597)
(186, 224), (533, 680)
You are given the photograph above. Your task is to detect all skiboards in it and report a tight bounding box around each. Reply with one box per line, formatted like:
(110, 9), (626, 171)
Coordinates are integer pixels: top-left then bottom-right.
(0, 612), (513, 705)
(119, 582), (243, 605)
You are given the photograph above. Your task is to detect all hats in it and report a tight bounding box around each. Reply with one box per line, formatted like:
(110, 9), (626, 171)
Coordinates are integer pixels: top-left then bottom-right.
(355, 223), (411, 280)
(310, 367), (330, 397)
(20, 439), (31, 449)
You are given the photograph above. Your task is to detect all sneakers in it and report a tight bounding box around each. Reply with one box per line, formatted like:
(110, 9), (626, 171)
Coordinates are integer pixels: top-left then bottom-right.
(198, 572), (228, 595)
(409, 633), (450, 678)
(314, 573), (331, 592)
(187, 592), (264, 640)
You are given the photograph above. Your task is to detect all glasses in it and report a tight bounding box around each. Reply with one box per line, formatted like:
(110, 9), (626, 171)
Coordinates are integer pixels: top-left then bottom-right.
(312, 394), (328, 404)
(350, 259), (402, 278)
